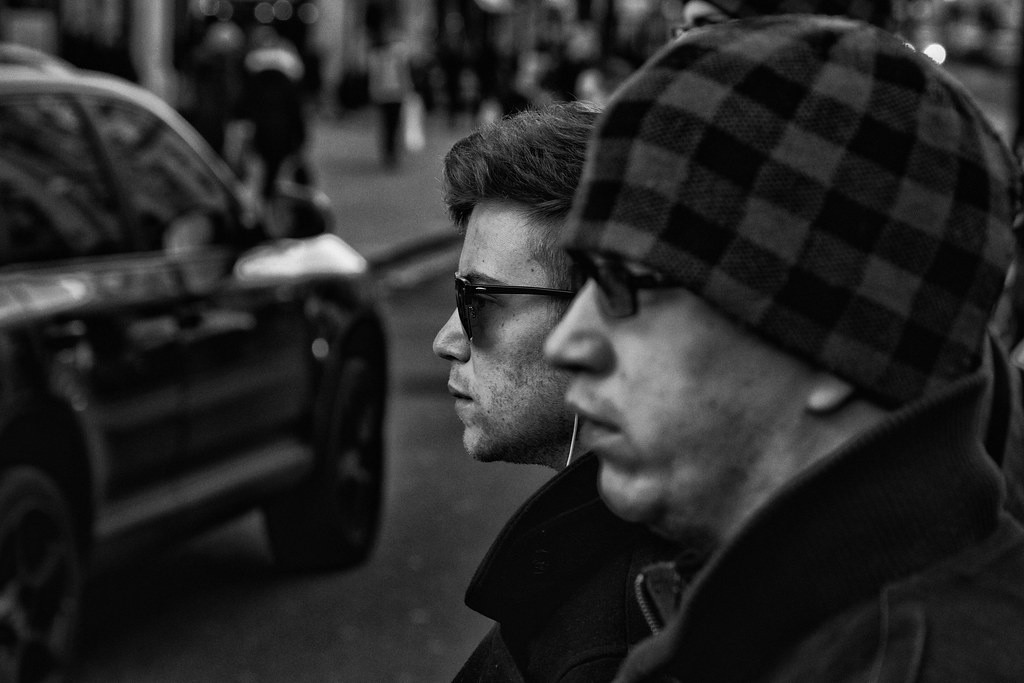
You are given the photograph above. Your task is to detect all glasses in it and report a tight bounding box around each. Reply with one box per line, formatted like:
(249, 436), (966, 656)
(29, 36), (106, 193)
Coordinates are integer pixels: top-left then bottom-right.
(564, 250), (680, 319)
(454, 275), (575, 343)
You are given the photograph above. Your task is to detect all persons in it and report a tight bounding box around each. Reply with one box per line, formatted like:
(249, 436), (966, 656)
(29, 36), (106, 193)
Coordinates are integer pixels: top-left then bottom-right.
(431, 105), (709, 683)
(56, 0), (1024, 214)
(544, 16), (1024, 682)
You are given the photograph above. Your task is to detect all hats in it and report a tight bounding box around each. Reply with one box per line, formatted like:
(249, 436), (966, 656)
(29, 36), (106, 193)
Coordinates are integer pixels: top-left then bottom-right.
(566, 14), (1020, 412)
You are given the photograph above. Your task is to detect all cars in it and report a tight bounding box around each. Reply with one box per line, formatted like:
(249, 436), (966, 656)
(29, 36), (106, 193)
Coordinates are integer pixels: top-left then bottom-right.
(0, 49), (390, 683)
